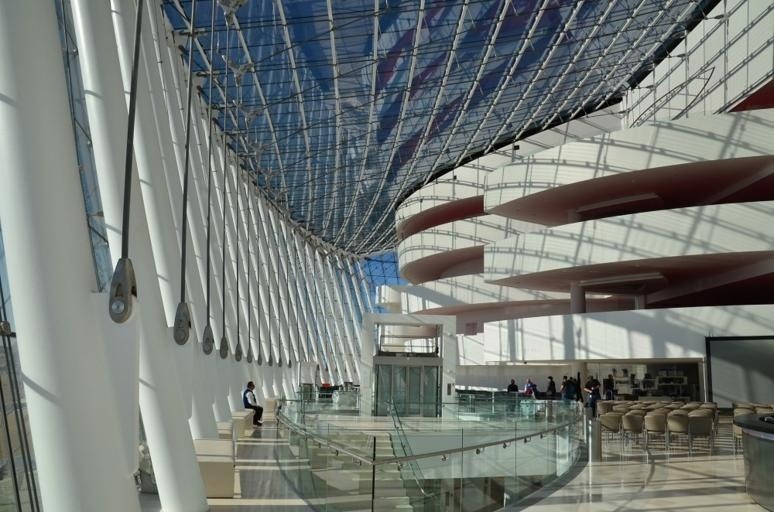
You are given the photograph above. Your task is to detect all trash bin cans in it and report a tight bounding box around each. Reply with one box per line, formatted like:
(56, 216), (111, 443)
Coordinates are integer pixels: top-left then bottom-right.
(302, 384), (312, 402)
(344, 382), (352, 393)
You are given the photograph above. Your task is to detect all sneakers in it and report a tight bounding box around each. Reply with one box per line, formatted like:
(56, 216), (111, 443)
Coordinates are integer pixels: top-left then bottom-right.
(253, 420), (262, 426)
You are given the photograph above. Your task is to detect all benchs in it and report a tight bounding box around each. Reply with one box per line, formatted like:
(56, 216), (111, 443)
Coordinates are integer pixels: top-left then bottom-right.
(175, 393), (284, 501)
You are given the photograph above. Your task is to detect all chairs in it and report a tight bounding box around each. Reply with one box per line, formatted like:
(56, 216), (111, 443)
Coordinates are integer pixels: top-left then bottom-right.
(586, 392), (774, 457)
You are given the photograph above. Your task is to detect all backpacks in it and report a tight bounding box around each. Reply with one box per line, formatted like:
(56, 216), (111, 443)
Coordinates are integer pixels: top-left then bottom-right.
(525, 383), (533, 396)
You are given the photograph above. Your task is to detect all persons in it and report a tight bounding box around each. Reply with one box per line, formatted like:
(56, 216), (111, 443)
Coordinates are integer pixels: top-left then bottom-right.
(507, 377), (519, 412)
(523, 370), (615, 421)
(243, 381), (263, 427)
